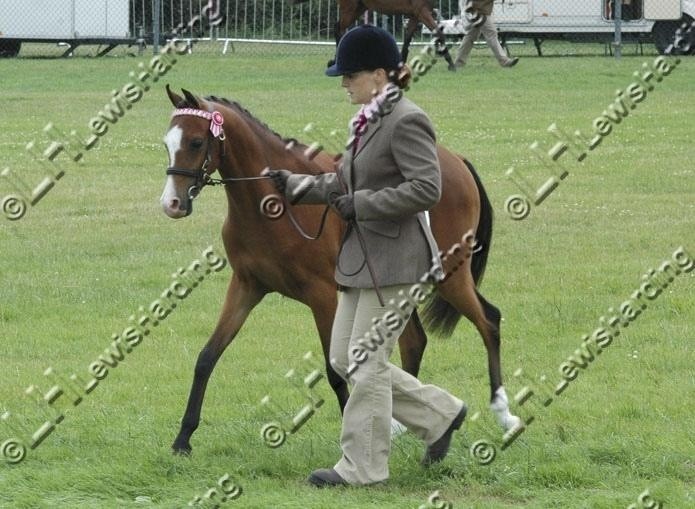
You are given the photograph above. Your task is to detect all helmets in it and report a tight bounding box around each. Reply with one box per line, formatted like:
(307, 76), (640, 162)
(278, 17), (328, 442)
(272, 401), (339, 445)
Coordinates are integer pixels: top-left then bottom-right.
(325, 25), (404, 77)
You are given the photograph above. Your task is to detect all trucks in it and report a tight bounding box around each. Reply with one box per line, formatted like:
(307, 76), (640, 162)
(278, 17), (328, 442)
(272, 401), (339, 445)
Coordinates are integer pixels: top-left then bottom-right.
(416, 0), (695, 56)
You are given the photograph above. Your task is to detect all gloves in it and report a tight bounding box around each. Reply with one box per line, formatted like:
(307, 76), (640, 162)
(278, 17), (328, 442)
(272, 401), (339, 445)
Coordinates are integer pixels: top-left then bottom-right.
(263, 169), (292, 197)
(335, 195), (355, 220)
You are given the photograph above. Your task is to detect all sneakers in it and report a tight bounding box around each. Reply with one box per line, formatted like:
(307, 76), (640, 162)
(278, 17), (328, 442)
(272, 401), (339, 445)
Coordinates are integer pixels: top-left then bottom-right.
(423, 403), (467, 467)
(506, 57), (518, 67)
(309, 468), (343, 488)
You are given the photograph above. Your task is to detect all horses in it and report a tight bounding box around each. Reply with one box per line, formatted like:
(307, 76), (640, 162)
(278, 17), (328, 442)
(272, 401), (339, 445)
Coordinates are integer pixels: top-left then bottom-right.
(159, 83), (523, 458)
(290, 0), (455, 71)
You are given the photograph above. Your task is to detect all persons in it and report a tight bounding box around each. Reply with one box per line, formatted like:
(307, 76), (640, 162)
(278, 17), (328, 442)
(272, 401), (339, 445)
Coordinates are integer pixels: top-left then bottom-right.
(454, 0), (519, 67)
(268, 23), (468, 489)
